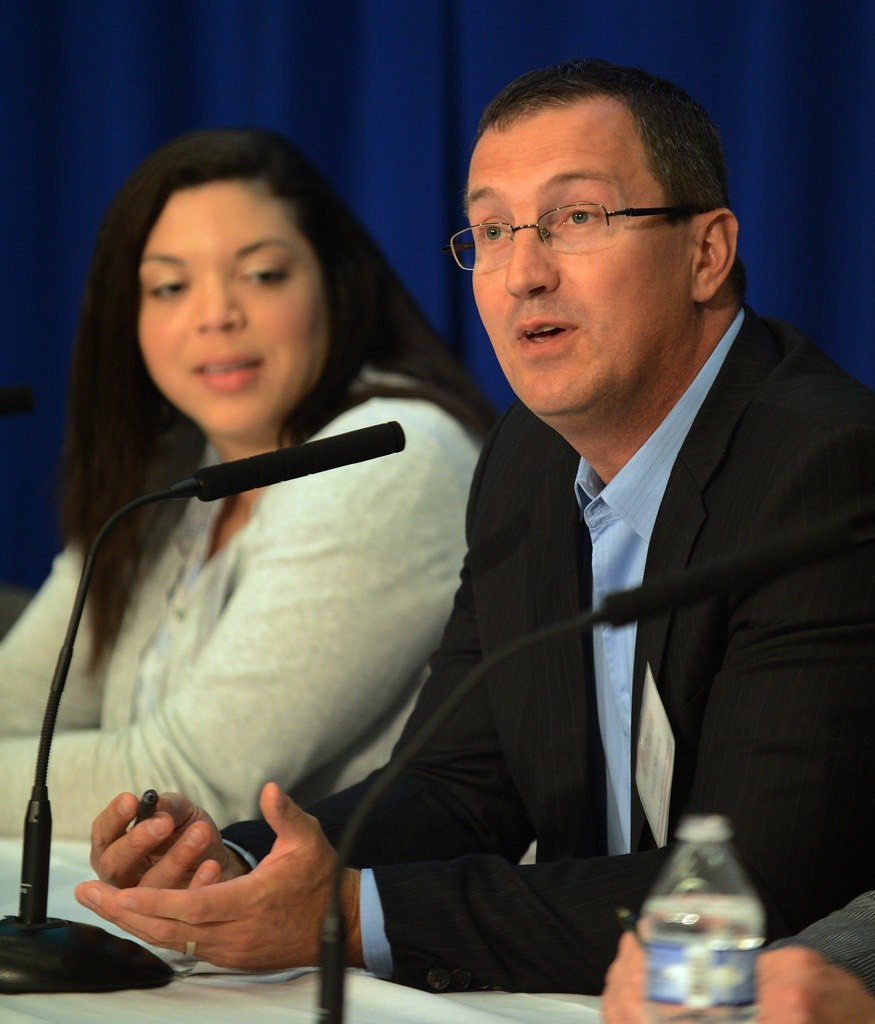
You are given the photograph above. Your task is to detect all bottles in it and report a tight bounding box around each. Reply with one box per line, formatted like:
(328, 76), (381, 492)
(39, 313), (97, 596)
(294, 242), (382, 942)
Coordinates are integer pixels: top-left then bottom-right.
(637, 813), (766, 1024)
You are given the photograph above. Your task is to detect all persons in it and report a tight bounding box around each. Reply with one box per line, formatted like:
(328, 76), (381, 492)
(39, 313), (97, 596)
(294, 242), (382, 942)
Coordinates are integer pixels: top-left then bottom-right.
(73, 59), (875, 1024)
(600, 875), (875, 1024)
(0, 121), (486, 844)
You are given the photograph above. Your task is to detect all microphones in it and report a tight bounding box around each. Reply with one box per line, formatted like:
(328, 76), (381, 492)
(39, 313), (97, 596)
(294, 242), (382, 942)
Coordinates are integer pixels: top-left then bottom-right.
(0, 421), (406, 994)
(316, 505), (875, 1024)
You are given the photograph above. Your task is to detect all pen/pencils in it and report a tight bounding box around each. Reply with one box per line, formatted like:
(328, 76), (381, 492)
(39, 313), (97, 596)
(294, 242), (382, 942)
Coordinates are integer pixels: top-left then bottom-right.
(131, 789), (159, 828)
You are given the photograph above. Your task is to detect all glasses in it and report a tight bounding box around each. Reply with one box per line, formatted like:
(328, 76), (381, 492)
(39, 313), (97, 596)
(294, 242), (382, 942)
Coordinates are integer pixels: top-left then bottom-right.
(441, 202), (719, 273)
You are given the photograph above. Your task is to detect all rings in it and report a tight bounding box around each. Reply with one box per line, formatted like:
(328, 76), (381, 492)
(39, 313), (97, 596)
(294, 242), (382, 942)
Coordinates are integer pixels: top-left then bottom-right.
(183, 941), (196, 957)
